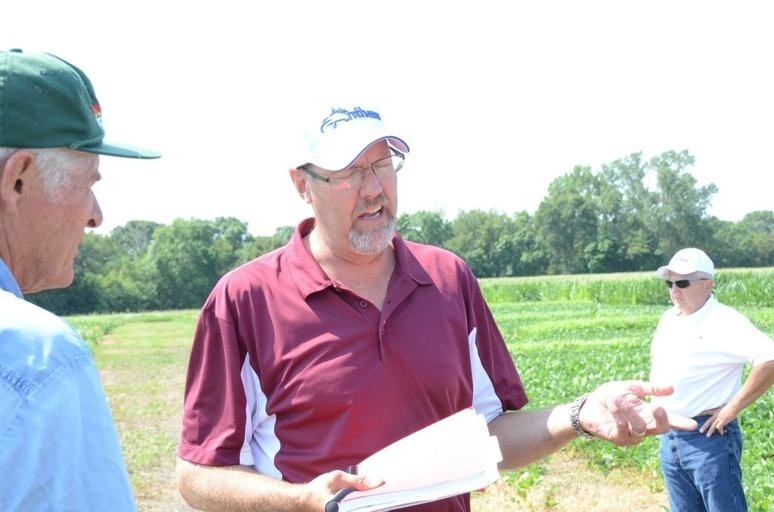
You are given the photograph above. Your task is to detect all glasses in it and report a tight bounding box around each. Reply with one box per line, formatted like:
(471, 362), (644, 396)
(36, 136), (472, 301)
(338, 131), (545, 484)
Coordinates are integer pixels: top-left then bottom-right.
(299, 146), (405, 190)
(665, 277), (709, 289)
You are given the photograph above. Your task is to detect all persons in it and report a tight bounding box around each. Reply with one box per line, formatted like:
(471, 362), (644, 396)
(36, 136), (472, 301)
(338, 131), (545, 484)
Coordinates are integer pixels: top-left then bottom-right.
(649, 247), (774, 512)
(176, 96), (699, 512)
(1, 45), (160, 512)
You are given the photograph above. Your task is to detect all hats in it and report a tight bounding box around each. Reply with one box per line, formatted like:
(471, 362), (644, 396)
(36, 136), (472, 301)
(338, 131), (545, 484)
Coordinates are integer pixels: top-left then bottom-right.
(655, 247), (715, 278)
(0, 49), (162, 160)
(288, 99), (409, 174)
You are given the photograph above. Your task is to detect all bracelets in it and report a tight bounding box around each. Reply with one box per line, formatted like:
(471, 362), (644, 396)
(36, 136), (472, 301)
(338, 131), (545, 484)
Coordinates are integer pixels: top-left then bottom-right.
(571, 393), (594, 440)
